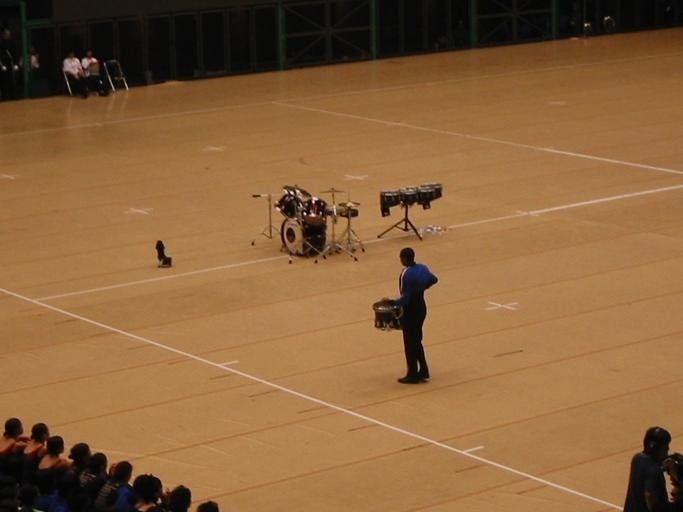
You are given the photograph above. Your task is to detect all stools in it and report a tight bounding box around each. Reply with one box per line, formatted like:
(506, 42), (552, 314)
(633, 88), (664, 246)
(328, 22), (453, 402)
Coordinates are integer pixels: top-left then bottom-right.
(84, 73), (103, 94)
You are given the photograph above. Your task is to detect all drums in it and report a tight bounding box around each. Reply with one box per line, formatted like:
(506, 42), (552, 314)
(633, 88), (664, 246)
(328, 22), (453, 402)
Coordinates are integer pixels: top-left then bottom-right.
(402, 187), (417, 204)
(383, 192), (400, 206)
(419, 184), (441, 201)
(278, 195), (310, 218)
(304, 198), (325, 225)
(281, 215), (326, 255)
(373, 301), (401, 329)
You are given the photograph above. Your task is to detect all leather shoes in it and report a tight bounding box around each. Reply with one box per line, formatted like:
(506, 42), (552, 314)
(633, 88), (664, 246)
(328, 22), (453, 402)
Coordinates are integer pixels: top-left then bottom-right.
(397, 373), (430, 384)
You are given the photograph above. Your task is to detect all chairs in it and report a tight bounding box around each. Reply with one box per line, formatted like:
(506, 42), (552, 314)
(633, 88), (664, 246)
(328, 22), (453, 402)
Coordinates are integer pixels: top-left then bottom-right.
(103, 58), (130, 93)
(61, 66), (81, 96)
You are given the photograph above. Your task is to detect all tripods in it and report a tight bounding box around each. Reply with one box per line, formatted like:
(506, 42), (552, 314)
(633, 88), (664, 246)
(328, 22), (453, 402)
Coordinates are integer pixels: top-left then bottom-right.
(250, 189), (366, 264)
(377, 204), (422, 240)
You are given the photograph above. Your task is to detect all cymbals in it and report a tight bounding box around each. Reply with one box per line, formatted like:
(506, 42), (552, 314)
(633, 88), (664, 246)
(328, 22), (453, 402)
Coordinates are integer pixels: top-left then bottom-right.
(339, 201), (359, 207)
(282, 186), (311, 199)
(321, 190), (342, 192)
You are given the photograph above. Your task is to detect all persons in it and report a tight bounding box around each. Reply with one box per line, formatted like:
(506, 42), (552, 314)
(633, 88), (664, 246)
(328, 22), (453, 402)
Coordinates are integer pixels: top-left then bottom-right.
(623, 427), (671, 511)
(382, 247), (438, 383)
(0, 417), (226, 510)
(0, 27), (109, 99)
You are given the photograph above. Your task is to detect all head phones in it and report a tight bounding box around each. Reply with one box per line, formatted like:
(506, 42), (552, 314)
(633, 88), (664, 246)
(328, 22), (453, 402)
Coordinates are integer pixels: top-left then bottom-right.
(648, 427), (662, 452)
